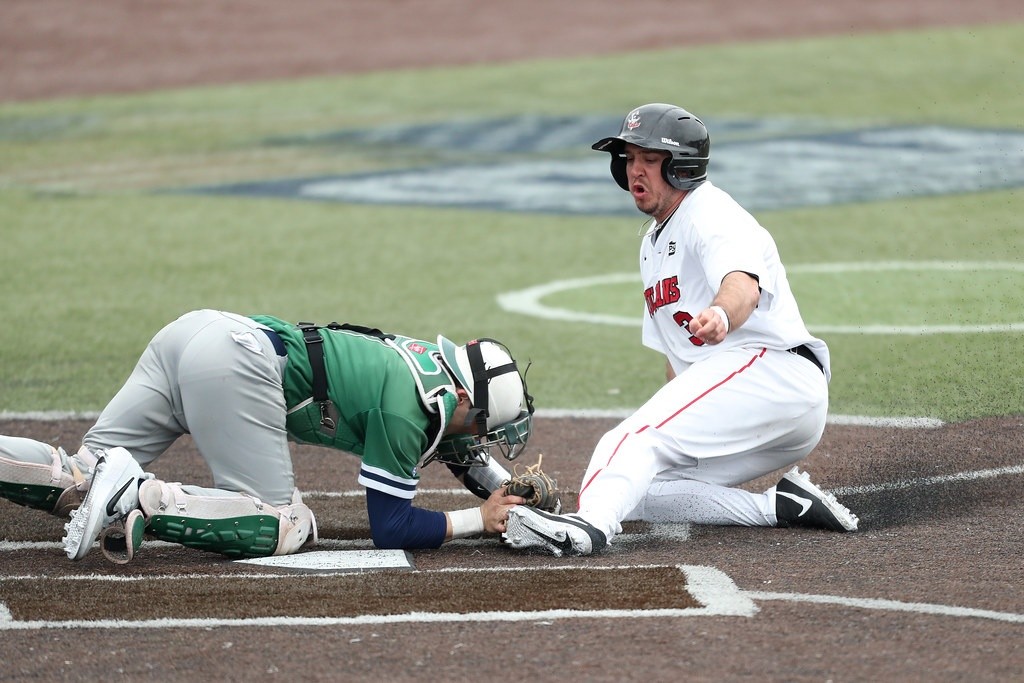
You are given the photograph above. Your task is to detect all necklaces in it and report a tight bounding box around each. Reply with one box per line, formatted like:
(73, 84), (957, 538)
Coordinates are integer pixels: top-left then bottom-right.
(638, 204), (680, 236)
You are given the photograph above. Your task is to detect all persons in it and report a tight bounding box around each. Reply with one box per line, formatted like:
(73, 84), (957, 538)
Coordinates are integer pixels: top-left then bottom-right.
(501, 102), (862, 556)
(0, 309), (564, 564)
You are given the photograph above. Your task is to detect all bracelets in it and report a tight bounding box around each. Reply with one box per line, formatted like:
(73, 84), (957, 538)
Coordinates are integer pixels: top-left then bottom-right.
(709, 306), (731, 334)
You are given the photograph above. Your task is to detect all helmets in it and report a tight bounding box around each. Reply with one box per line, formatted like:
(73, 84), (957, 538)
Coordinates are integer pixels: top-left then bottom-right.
(437, 334), (524, 432)
(591, 103), (711, 190)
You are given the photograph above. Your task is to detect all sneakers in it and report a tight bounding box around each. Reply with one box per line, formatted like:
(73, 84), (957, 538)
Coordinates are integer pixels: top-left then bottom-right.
(776, 465), (860, 532)
(502, 505), (607, 557)
(62, 447), (146, 560)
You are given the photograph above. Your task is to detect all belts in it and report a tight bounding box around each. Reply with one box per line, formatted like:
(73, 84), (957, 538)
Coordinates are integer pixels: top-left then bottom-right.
(261, 329), (287, 357)
(785, 344), (824, 374)
